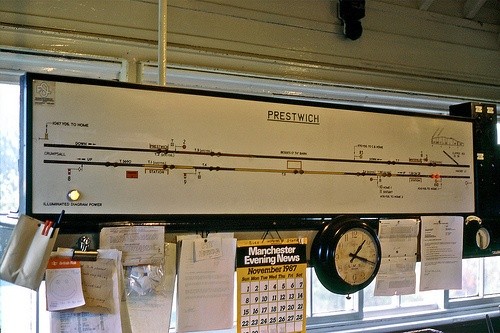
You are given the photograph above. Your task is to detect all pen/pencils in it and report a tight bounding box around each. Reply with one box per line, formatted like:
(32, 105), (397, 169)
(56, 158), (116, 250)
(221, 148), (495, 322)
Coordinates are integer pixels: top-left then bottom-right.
(44, 221), (52, 235)
(57, 209), (65, 228)
(41, 220), (49, 235)
(50, 221), (58, 238)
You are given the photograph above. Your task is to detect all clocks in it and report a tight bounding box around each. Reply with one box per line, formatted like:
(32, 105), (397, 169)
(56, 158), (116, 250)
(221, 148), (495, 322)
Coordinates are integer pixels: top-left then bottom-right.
(311, 219), (381, 300)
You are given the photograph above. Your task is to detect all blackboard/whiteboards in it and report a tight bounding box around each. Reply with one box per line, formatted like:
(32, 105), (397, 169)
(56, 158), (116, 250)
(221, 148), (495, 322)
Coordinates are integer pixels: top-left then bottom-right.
(24, 72), (485, 234)
